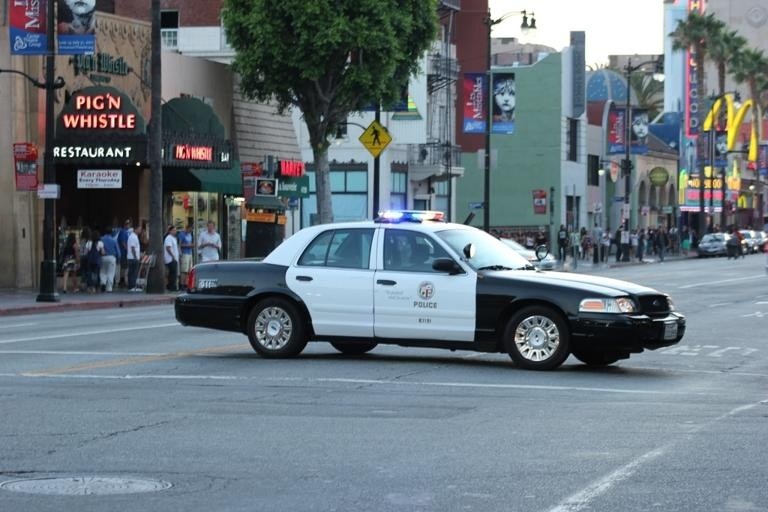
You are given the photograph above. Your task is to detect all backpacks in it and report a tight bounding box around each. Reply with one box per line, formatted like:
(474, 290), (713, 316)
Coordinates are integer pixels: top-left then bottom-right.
(87, 239), (102, 273)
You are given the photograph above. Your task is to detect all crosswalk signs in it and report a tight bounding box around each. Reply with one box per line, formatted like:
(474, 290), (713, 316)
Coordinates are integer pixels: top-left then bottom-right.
(358, 120), (392, 159)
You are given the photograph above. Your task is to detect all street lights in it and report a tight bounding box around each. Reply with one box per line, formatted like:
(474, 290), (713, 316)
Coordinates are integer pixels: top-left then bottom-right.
(708, 89), (743, 231)
(485, 8), (537, 233)
(598, 58), (665, 262)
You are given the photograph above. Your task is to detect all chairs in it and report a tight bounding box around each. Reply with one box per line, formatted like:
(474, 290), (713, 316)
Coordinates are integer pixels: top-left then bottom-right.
(390, 245), (435, 267)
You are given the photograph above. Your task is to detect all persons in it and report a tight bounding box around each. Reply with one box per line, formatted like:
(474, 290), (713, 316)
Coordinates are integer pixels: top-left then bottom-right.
(632, 114), (650, 142)
(59, 0), (95, 36)
(61, 219), (222, 293)
(535, 232), (548, 245)
(494, 80), (516, 119)
(523, 231), (535, 249)
(715, 135), (727, 162)
(559, 223), (754, 262)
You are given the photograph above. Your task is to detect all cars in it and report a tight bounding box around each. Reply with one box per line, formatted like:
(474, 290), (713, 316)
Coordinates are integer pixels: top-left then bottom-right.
(496, 237), (557, 271)
(697, 230), (768, 257)
(175, 210), (686, 369)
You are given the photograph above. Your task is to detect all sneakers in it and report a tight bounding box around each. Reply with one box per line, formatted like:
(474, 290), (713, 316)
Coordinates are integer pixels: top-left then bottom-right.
(127, 287), (143, 293)
